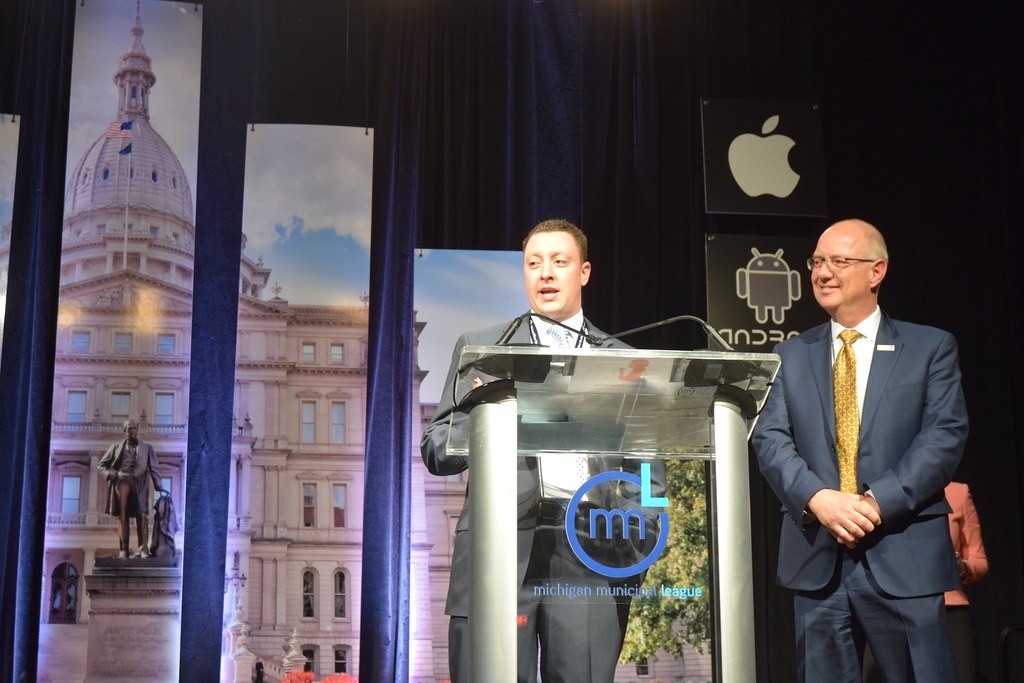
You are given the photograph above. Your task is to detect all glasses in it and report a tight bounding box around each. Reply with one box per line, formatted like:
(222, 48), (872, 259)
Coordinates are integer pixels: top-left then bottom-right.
(807, 255), (874, 271)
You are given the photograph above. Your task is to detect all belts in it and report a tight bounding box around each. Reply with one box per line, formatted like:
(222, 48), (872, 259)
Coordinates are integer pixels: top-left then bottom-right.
(540, 497), (589, 526)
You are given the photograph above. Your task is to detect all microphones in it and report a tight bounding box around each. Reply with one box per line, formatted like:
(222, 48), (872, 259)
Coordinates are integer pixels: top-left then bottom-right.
(473, 313), (759, 387)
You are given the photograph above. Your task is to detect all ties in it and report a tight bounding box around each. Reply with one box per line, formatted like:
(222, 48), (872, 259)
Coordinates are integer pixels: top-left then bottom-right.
(831, 329), (860, 495)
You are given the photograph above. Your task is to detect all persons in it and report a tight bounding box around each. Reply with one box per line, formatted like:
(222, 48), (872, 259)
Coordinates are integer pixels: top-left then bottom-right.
(750, 219), (966, 683)
(421, 220), (668, 683)
(96, 418), (161, 559)
(943, 482), (989, 683)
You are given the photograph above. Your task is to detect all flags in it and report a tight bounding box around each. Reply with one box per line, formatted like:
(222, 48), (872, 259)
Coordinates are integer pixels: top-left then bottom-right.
(105, 121), (132, 137)
